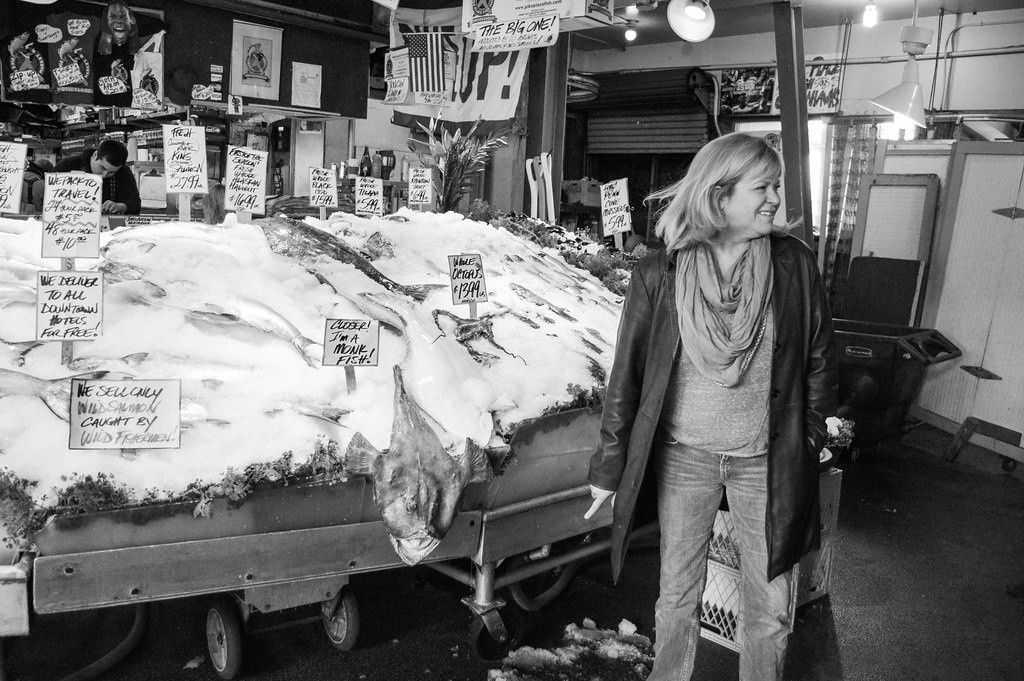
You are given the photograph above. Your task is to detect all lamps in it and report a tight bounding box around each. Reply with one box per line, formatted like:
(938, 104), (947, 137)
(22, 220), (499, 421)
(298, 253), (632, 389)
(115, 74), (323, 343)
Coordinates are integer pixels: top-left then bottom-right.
(868, 0), (933, 129)
(624, 18), (639, 42)
(667, 0), (714, 43)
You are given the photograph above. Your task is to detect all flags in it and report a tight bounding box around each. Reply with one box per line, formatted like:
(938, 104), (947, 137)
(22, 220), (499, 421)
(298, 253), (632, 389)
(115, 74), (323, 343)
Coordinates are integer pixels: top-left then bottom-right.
(409, 32), (446, 93)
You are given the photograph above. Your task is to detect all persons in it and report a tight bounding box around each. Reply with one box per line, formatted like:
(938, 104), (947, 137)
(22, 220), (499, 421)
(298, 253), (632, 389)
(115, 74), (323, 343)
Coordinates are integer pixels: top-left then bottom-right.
(95, 3), (142, 105)
(204, 185), (229, 224)
(55, 140), (141, 216)
(583, 133), (838, 681)
(22, 160), (53, 210)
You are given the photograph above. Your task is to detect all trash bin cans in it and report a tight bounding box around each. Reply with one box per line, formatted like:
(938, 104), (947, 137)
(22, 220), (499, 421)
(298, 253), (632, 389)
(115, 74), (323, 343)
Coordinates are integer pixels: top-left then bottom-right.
(831, 316), (964, 505)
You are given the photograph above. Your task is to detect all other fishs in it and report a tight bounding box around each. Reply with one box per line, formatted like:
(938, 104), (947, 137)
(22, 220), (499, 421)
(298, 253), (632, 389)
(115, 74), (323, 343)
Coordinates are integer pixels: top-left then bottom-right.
(0, 215), (623, 429)
(344, 363), (494, 567)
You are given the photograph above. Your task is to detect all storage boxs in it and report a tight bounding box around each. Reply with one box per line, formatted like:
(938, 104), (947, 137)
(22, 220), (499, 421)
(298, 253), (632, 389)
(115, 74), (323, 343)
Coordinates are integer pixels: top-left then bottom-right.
(700, 470), (842, 652)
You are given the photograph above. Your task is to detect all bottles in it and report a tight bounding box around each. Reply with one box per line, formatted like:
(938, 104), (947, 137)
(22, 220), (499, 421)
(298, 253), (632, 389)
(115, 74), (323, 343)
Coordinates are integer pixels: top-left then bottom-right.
(360, 145), (371, 176)
(371, 151), (382, 178)
(401, 156), (410, 182)
